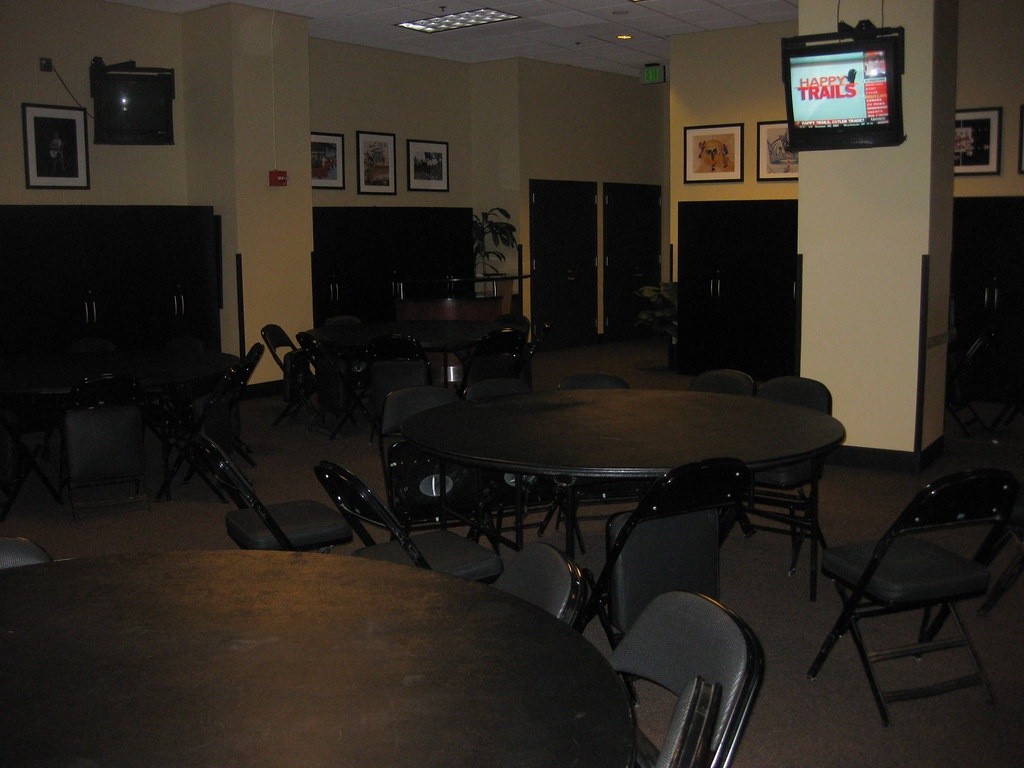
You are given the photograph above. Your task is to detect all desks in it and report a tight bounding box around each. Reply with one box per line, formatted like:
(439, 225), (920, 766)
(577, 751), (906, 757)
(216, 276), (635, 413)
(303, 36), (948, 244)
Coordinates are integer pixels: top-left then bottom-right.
(400, 389), (845, 603)
(0, 549), (636, 768)
(393, 273), (531, 382)
(0, 353), (240, 490)
(307, 320), (530, 388)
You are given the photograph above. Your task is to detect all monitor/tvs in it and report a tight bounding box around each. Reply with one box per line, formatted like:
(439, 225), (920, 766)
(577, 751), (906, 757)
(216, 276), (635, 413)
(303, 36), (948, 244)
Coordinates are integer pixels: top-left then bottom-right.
(94, 74), (175, 145)
(782, 37), (903, 152)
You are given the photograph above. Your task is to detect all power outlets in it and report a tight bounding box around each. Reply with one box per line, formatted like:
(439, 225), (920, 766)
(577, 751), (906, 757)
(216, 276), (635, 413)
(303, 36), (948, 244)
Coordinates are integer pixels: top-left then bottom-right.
(40, 57), (53, 73)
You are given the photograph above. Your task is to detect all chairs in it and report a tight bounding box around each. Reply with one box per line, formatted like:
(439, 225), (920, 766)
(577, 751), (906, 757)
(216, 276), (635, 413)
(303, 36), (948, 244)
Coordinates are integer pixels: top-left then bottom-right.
(0, 313), (1024, 768)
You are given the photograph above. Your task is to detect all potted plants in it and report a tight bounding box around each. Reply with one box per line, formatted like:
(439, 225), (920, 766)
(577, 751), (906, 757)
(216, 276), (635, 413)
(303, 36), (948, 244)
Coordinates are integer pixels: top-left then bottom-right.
(635, 282), (678, 371)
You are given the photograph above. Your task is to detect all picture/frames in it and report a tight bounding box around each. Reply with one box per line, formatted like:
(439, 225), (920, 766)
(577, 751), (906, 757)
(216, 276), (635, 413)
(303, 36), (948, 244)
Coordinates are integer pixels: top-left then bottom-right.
(20, 101), (91, 190)
(311, 130), (346, 189)
(406, 139), (449, 192)
(683, 122), (744, 183)
(756, 121), (799, 180)
(355, 130), (396, 195)
(954, 105), (1003, 176)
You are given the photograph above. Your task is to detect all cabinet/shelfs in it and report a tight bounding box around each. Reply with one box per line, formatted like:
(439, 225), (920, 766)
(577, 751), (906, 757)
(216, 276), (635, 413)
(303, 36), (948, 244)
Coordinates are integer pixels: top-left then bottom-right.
(952, 198), (1024, 407)
(312, 206), (476, 358)
(674, 199), (798, 380)
(0, 205), (222, 429)
